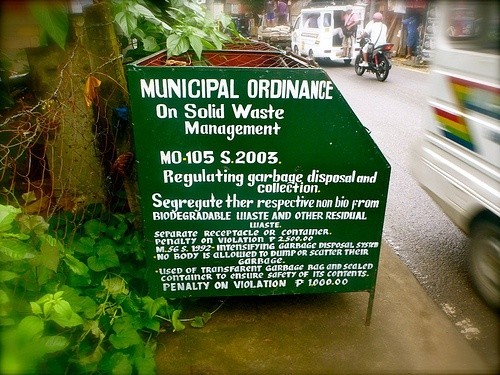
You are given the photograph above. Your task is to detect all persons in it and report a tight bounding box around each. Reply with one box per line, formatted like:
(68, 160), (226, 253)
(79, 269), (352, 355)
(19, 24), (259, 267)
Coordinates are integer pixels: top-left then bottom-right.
(341, 4), (359, 57)
(402, 11), (416, 59)
(274, 0), (287, 25)
(304, 14), (314, 28)
(23, 80), (135, 235)
(265, 1), (273, 23)
(365, 19), (374, 29)
(359, 13), (387, 67)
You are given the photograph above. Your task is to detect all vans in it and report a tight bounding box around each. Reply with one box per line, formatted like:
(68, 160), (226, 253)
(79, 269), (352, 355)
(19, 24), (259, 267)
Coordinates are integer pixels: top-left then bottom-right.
(405, 0), (500, 314)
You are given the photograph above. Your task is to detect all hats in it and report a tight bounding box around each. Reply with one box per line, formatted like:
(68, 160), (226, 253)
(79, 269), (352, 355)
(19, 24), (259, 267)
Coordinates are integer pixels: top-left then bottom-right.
(372, 12), (383, 19)
(344, 5), (353, 12)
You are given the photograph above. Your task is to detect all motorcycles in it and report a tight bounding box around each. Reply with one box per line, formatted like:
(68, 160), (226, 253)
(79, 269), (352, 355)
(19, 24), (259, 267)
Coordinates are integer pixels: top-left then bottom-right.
(355, 31), (394, 81)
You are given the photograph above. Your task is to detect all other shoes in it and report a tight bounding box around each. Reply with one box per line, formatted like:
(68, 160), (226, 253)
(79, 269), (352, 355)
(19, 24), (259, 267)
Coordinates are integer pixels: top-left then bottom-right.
(340, 53), (351, 58)
(358, 61), (370, 67)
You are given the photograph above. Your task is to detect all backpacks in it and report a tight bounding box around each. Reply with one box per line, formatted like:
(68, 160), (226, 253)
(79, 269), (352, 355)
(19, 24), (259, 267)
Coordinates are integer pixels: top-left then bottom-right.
(341, 24), (357, 38)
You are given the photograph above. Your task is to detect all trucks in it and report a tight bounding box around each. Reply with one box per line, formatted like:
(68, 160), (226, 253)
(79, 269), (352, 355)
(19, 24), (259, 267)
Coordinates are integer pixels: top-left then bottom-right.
(290, 5), (366, 64)
(257, 15), (293, 50)
